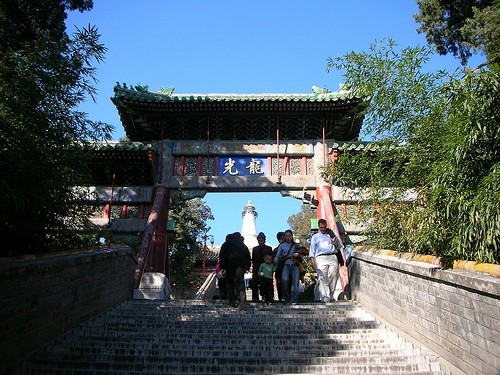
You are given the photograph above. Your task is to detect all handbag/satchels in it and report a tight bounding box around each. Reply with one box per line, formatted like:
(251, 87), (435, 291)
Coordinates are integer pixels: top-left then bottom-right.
(276, 262), (284, 279)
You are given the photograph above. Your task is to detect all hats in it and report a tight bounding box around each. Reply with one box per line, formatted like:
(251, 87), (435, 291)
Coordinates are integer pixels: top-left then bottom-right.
(257, 232), (266, 239)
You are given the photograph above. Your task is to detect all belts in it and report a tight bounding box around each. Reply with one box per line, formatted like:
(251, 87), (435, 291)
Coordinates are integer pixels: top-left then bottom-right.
(319, 253), (335, 256)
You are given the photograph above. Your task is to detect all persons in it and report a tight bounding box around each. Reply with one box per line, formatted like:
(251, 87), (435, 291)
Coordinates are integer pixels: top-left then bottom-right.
(308, 219), (343, 302)
(216, 232), (253, 307)
(251, 232), (273, 302)
(272, 230), (301, 305)
(258, 254), (278, 305)
